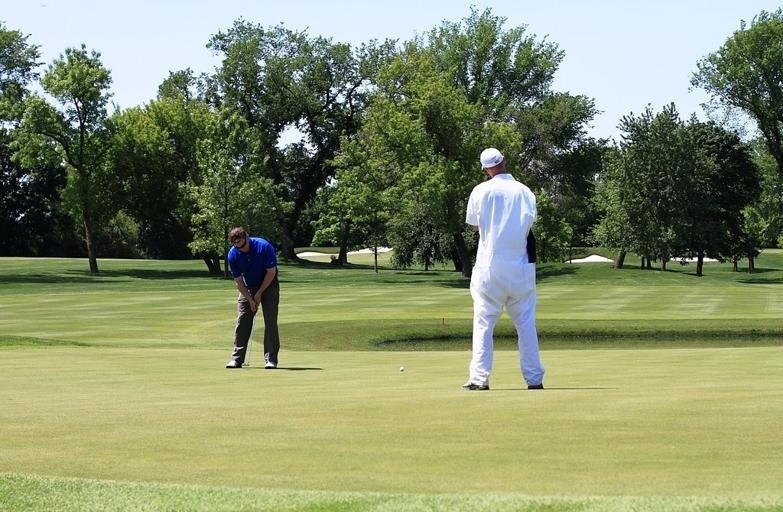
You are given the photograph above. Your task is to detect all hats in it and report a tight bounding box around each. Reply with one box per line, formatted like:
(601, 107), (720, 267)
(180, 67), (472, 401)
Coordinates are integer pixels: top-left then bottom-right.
(480, 148), (505, 170)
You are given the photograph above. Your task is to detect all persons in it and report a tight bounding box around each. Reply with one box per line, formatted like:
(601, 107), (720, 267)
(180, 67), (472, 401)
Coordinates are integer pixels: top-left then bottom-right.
(226, 226), (281, 369)
(462, 147), (545, 390)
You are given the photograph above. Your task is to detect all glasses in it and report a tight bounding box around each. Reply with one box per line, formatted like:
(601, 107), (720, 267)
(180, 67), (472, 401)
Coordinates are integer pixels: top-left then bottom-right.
(235, 237), (247, 249)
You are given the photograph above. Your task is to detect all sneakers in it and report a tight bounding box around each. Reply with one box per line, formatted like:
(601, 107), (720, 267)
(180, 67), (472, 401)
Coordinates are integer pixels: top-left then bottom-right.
(528, 382), (544, 390)
(462, 382), (490, 391)
(226, 360), (243, 368)
(265, 361), (278, 368)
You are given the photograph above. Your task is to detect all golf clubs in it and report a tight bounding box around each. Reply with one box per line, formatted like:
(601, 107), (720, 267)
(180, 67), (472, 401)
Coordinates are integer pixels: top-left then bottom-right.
(243, 313), (255, 367)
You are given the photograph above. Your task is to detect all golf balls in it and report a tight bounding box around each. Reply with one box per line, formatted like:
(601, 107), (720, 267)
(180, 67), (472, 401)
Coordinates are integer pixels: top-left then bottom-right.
(400, 367), (404, 371)
